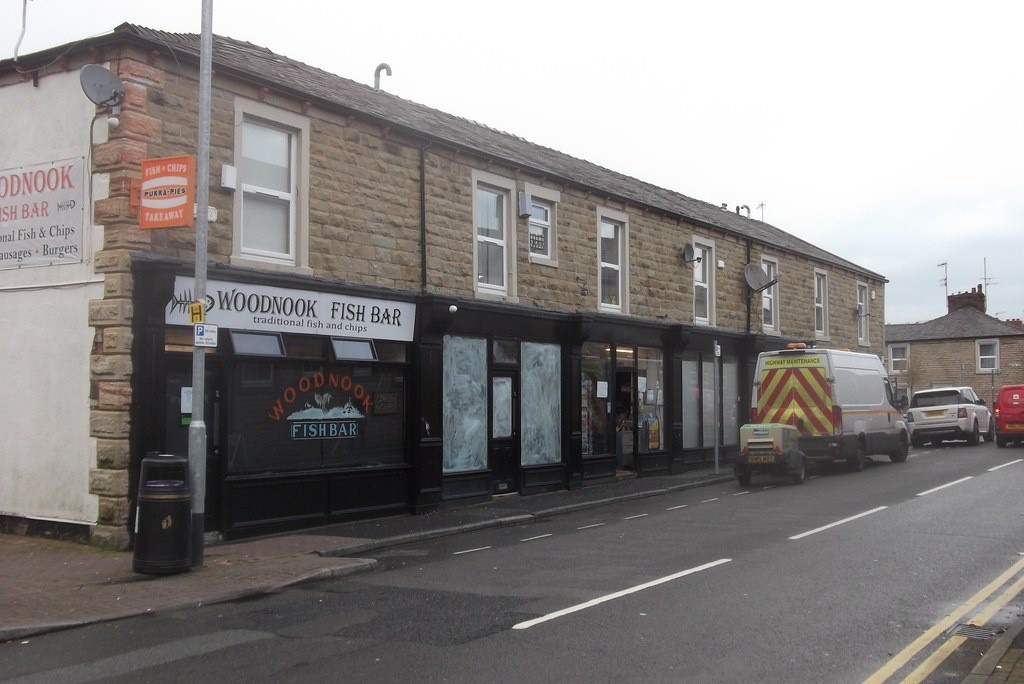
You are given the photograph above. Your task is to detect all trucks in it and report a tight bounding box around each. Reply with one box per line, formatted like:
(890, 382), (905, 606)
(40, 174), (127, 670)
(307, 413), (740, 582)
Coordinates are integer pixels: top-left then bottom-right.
(751, 341), (911, 473)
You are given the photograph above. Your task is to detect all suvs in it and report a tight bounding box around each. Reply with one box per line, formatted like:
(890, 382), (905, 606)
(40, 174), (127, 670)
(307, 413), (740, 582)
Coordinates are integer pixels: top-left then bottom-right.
(907, 385), (994, 447)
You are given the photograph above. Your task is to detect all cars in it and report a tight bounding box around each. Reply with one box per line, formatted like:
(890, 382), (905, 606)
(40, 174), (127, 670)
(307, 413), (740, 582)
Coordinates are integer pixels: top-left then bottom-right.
(733, 421), (807, 487)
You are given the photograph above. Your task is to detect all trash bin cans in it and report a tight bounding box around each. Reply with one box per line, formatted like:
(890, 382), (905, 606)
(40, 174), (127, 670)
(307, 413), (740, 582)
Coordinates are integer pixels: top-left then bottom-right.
(130, 454), (194, 576)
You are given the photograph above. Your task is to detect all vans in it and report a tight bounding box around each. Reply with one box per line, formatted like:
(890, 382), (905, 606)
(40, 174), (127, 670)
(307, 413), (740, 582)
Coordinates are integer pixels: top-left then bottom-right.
(996, 385), (1024, 449)
